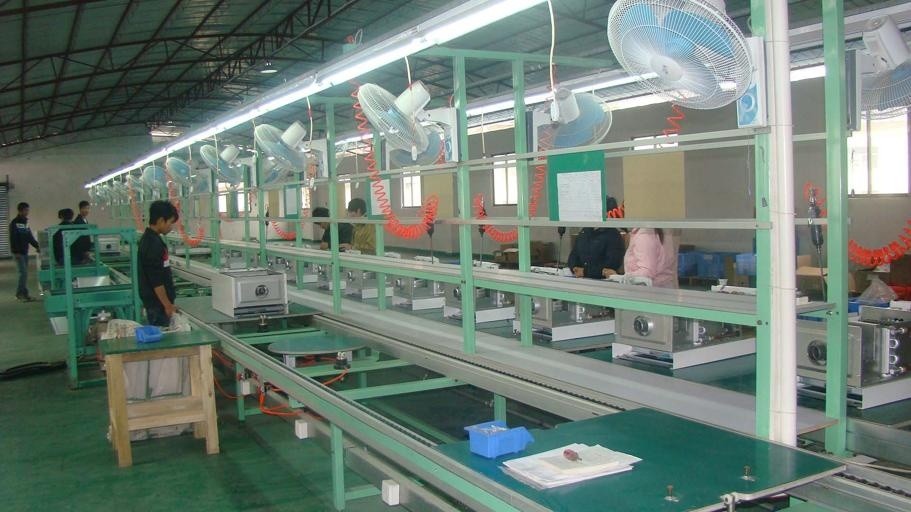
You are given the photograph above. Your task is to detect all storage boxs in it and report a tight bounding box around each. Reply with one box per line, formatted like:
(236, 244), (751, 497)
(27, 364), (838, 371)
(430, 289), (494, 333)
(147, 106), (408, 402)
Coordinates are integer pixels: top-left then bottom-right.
(796, 254), (910, 292)
(725, 256), (749, 287)
(494, 241), (553, 264)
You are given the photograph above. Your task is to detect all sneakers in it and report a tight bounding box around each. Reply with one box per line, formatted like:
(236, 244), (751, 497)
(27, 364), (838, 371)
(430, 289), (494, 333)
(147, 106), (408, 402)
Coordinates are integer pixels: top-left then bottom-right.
(14, 292), (36, 303)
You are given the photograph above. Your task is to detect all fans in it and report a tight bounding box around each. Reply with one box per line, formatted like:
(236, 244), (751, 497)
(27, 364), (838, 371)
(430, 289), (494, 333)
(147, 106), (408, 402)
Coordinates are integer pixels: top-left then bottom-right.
(607, 1), (767, 128)
(858, 14), (909, 117)
(527, 87), (613, 200)
(254, 98), (314, 200)
(351, 58), (445, 201)
(87, 137), (242, 199)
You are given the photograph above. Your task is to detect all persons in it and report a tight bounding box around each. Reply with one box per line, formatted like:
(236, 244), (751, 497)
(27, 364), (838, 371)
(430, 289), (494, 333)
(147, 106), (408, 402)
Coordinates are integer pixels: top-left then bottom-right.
(54, 209), (82, 265)
(58, 210), (63, 218)
(313, 207), (354, 250)
(75, 201), (91, 251)
(605, 227), (677, 289)
(137, 201), (180, 328)
(10, 202), (40, 302)
(569, 196), (624, 279)
(340, 196), (376, 252)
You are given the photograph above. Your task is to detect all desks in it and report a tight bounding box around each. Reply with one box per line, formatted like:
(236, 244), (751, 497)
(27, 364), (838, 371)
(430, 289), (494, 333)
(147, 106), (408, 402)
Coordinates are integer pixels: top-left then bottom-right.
(98, 329), (222, 470)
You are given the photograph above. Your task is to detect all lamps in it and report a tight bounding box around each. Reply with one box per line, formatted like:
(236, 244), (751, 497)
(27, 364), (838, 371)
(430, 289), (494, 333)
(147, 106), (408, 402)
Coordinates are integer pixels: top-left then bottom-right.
(789, 57), (827, 84)
(192, 2), (545, 134)
(546, 47), (698, 114)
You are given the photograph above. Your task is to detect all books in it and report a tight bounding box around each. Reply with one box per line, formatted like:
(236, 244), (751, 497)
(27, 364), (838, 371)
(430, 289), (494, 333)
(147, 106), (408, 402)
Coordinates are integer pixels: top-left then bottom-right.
(498, 439), (644, 490)
